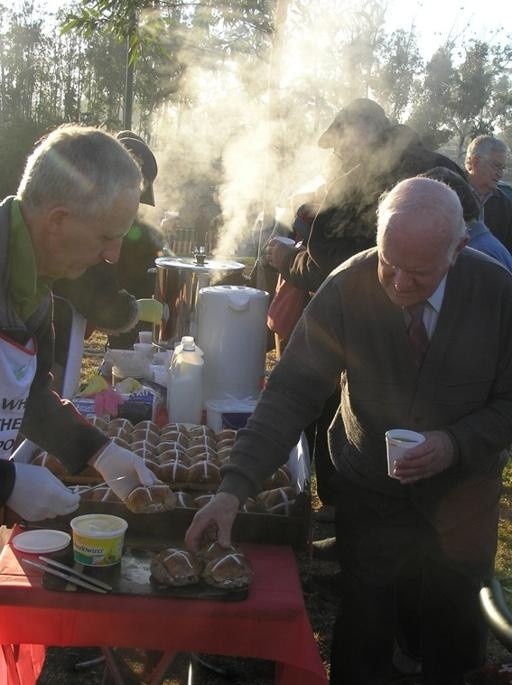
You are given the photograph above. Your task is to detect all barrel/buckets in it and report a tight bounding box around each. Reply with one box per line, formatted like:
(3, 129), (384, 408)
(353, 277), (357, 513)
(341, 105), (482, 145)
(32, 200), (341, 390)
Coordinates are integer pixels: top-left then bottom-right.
(170, 336), (204, 377)
(168, 344), (205, 423)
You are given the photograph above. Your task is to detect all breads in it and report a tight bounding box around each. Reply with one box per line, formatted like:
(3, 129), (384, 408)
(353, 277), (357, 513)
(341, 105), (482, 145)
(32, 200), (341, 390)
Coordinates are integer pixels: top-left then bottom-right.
(32, 415), (297, 589)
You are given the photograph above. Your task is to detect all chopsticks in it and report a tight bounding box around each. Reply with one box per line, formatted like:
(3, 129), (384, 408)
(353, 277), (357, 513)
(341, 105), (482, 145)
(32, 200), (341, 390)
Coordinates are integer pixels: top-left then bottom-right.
(21, 555), (112, 594)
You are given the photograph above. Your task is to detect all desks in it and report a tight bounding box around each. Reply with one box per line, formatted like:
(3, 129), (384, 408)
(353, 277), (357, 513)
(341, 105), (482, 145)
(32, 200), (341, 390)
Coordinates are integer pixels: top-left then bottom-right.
(0, 429), (327, 685)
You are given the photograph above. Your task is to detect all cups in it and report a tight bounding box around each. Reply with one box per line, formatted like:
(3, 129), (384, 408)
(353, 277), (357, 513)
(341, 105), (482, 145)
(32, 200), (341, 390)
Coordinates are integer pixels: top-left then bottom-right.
(134, 331), (153, 360)
(386, 428), (425, 480)
(71, 513), (128, 567)
(272, 236), (296, 248)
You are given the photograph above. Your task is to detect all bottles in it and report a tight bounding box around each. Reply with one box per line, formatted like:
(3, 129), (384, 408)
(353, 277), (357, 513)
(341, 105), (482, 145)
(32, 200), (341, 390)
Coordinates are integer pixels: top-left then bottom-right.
(168, 336), (204, 425)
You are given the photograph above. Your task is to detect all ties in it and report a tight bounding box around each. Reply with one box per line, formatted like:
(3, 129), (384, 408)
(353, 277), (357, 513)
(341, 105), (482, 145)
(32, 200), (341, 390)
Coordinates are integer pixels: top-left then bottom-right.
(406, 304), (430, 371)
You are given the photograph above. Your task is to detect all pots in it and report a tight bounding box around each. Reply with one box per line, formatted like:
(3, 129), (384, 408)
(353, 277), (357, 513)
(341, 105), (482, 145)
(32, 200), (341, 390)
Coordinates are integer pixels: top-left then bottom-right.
(147, 249), (245, 351)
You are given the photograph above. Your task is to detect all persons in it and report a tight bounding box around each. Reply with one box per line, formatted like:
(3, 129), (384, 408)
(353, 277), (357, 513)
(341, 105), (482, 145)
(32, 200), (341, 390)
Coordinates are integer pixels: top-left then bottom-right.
(184, 177), (512, 685)
(2, 123), (161, 521)
(252, 99), (512, 459)
(53, 131), (166, 398)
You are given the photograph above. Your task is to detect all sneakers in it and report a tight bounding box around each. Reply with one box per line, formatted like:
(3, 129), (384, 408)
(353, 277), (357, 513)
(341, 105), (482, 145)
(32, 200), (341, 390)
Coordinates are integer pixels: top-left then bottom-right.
(310, 505), (341, 559)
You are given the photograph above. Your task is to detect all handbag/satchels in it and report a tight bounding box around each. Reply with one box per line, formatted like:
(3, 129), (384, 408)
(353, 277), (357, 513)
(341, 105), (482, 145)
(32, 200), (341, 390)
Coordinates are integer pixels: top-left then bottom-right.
(266, 278), (306, 341)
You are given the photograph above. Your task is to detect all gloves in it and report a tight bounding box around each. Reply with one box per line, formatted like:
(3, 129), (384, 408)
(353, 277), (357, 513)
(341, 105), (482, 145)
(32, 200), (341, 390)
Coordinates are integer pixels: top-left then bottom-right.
(93, 441), (157, 500)
(5, 462), (81, 521)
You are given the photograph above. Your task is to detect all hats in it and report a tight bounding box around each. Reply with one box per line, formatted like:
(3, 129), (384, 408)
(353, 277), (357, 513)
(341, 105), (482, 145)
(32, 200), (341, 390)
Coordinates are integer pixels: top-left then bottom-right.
(115, 130), (157, 207)
(317, 98), (390, 147)
